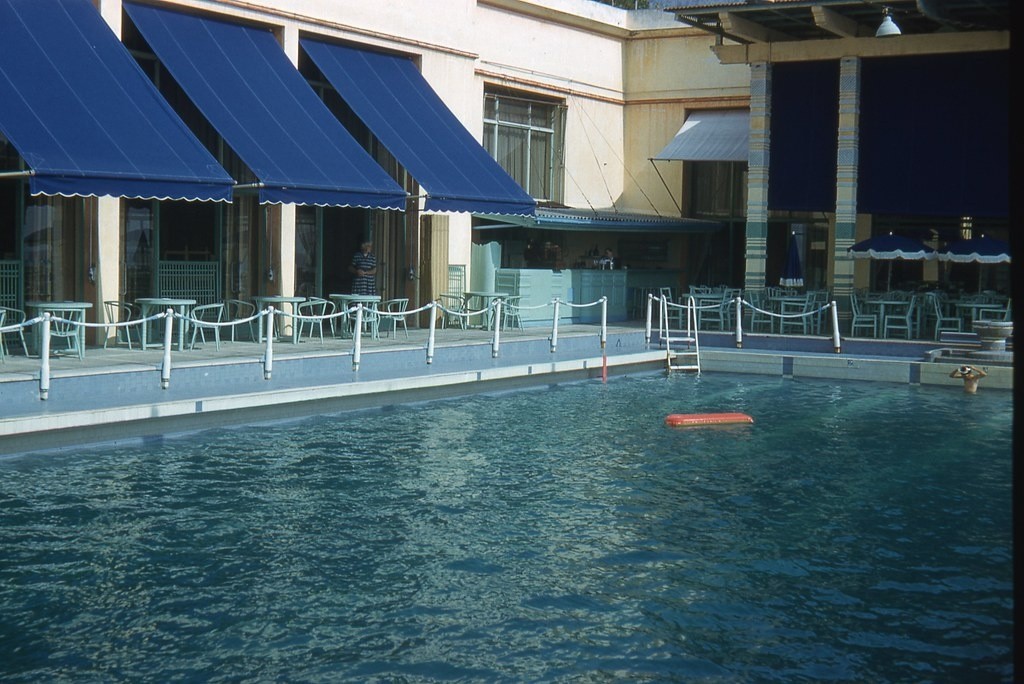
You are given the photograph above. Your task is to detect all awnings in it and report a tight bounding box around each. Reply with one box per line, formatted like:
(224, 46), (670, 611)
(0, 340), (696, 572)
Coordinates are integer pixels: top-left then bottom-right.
(654, 107), (748, 162)
(0, 0), (236, 203)
(471, 202), (726, 232)
(123, 3), (410, 212)
(300, 35), (538, 219)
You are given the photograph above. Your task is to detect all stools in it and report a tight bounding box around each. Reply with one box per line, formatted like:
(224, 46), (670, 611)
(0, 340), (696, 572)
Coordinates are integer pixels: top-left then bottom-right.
(632, 285), (687, 320)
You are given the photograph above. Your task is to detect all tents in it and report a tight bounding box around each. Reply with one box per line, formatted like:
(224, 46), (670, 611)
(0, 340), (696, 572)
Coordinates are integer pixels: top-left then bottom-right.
(848, 222), (935, 292)
(779, 231), (805, 287)
(938, 233), (1012, 304)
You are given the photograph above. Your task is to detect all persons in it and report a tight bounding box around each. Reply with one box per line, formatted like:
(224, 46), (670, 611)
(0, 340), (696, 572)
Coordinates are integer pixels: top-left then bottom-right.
(598, 248), (621, 270)
(950, 365), (986, 393)
(352, 236), (378, 332)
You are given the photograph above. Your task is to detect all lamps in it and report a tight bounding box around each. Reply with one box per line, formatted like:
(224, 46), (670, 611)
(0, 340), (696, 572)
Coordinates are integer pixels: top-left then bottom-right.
(876, 16), (901, 38)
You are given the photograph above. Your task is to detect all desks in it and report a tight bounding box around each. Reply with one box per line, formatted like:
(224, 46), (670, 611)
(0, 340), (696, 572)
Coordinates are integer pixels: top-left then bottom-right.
(768, 296), (807, 335)
(250, 296), (306, 345)
(464, 291), (509, 331)
(135, 298), (197, 352)
(955, 303), (1004, 333)
(682, 293), (722, 298)
(329, 294), (381, 339)
(26, 301), (94, 358)
(865, 300), (909, 339)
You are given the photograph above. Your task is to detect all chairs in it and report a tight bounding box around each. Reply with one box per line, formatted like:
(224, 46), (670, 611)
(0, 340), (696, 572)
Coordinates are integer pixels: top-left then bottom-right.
(660, 285), (1011, 341)
(0, 294), (524, 365)
